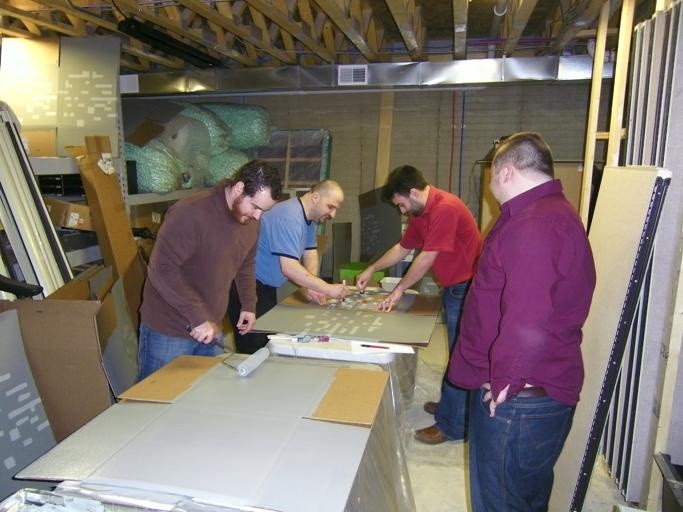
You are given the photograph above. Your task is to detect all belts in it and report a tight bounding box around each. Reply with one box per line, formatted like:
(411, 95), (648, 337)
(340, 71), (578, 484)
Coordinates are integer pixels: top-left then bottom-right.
(517, 386), (547, 398)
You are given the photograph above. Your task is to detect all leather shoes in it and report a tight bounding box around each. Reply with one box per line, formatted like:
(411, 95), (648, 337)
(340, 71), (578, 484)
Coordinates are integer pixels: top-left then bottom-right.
(413, 401), (448, 445)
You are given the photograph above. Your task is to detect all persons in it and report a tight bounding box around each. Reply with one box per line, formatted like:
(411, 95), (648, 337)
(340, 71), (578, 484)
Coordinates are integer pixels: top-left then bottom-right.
(354, 163), (485, 444)
(443, 131), (596, 511)
(131, 157), (284, 384)
(226, 179), (348, 332)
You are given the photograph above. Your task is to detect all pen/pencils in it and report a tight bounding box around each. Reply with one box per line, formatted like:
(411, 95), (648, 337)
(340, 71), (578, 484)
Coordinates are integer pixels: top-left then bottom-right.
(360, 290), (364, 294)
(343, 280), (345, 303)
(361, 345), (390, 350)
